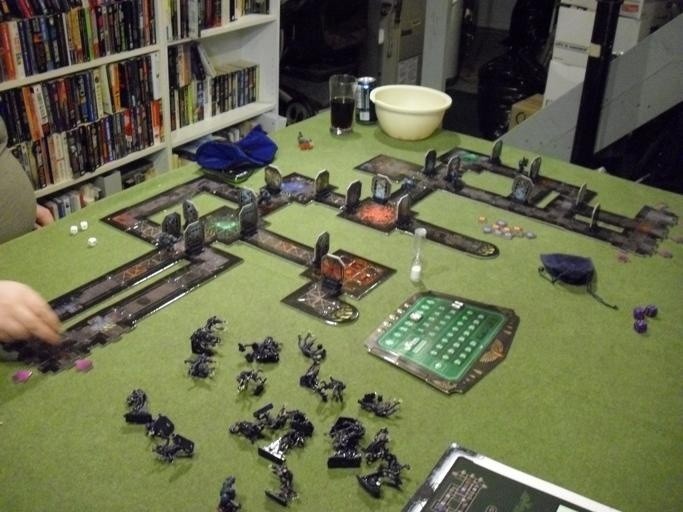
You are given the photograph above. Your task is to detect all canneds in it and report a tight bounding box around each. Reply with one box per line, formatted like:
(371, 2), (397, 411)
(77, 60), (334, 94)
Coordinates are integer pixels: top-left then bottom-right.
(353, 77), (377, 125)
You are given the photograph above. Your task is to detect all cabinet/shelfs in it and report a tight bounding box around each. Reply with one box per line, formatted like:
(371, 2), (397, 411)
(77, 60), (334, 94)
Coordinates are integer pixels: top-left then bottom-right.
(0, 0), (284, 241)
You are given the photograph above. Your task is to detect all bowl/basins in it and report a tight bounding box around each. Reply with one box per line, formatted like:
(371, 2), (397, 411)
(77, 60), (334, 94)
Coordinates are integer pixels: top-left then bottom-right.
(368, 84), (453, 142)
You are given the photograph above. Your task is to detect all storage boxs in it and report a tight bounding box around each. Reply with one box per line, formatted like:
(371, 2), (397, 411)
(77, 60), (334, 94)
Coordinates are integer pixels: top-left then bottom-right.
(504, 0), (676, 137)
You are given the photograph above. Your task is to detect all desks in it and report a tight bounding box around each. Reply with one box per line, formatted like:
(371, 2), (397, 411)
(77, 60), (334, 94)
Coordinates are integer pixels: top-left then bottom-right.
(1, 103), (680, 512)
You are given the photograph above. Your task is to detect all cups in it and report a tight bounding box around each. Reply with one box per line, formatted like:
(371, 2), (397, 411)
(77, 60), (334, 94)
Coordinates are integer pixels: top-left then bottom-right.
(328, 73), (357, 132)
